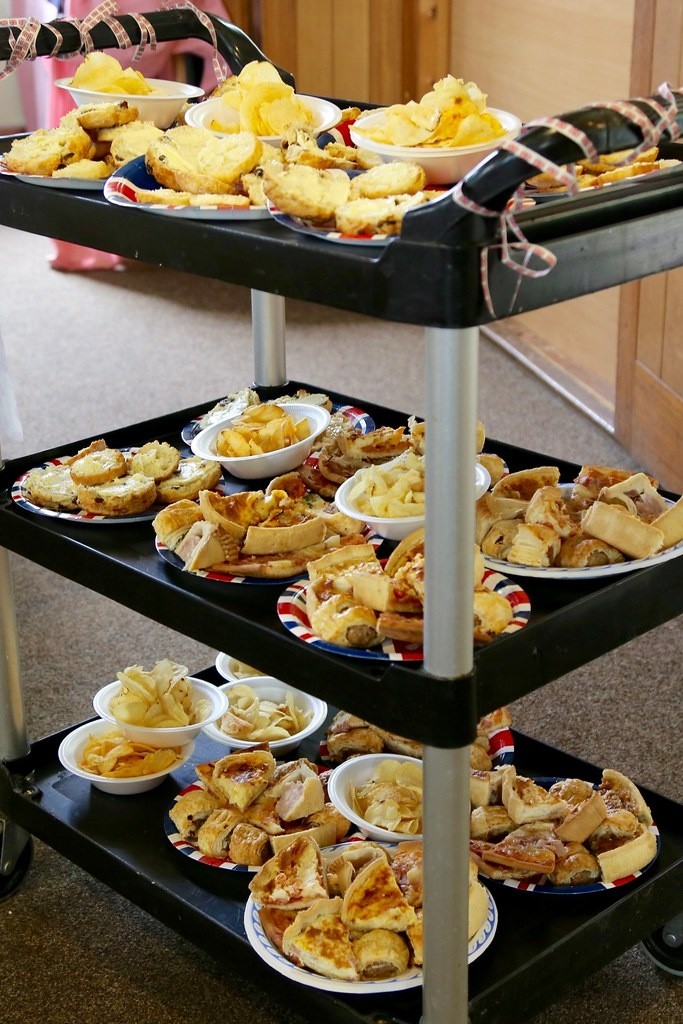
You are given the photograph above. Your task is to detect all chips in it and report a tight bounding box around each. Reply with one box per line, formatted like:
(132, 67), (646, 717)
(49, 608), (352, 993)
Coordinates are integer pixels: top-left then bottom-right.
(345, 73), (512, 149)
(77, 657), (302, 778)
(209, 60), (313, 138)
(70, 52), (157, 97)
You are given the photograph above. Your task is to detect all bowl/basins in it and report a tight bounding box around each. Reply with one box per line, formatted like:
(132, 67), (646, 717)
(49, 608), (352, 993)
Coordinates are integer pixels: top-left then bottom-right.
(53, 79), (206, 129)
(201, 676), (328, 753)
(185, 94), (343, 148)
(334, 456), (491, 539)
(327, 753), (423, 843)
(59, 719), (195, 794)
(93, 675), (229, 748)
(351, 106), (522, 187)
(190, 402), (332, 479)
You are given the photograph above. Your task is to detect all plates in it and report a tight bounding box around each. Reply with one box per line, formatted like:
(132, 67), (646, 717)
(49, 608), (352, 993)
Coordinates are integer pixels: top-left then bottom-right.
(10, 400), (683, 664)
(58, 651), (662, 995)
(0, 77), (683, 248)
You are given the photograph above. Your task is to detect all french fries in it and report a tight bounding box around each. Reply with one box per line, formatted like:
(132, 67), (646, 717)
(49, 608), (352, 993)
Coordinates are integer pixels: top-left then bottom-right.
(347, 451), (431, 518)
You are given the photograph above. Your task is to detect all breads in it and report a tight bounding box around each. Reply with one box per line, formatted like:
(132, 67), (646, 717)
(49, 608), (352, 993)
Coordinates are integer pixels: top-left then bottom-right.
(5, 100), (682, 234)
(24, 389), (332, 514)
(171, 711), (656, 981)
(153, 416), (683, 653)
